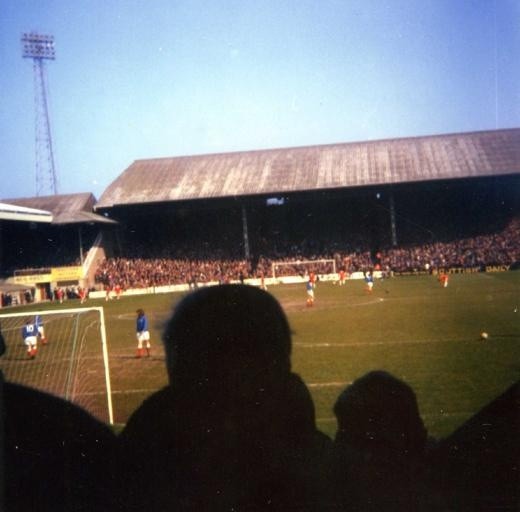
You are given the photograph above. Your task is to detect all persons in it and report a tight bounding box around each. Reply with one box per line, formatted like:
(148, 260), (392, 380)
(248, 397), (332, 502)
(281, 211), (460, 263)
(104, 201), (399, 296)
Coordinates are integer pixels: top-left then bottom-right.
(1, 201), (519, 511)
